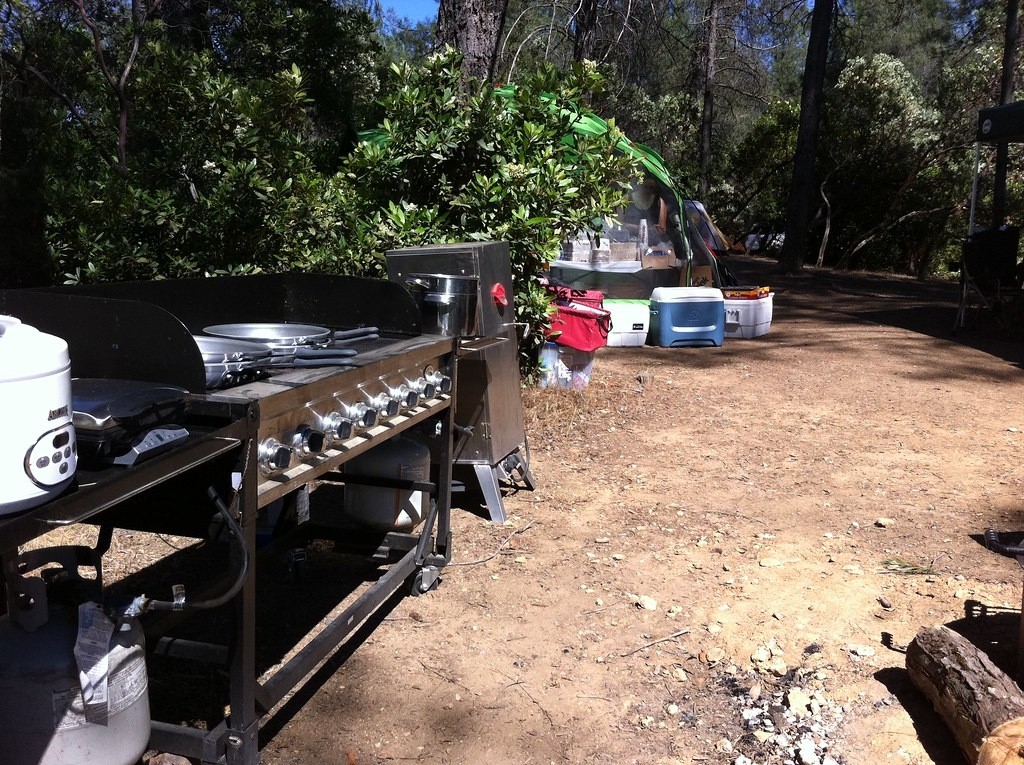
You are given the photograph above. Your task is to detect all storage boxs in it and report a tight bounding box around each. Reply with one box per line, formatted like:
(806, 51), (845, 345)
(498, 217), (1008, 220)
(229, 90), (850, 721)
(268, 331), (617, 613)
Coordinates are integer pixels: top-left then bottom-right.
(722, 286), (769, 300)
(561, 238), (610, 261)
(724, 292), (775, 339)
(610, 243), (637, 261)
(537, 340), (596, 391)
(649, 286), (724, 347)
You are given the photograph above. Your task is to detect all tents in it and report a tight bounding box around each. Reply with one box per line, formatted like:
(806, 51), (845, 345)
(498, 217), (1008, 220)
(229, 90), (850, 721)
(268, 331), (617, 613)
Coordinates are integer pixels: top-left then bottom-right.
(493, 82), (724, 290)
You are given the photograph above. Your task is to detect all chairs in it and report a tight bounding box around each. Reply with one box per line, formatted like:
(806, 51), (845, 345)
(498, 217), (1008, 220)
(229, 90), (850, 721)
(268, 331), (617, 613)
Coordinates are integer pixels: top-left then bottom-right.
(952, 227), (1024, 336)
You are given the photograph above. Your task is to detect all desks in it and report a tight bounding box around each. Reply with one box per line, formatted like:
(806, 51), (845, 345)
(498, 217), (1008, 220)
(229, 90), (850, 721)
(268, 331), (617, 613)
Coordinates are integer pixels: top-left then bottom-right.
(550, 258), (683, 300)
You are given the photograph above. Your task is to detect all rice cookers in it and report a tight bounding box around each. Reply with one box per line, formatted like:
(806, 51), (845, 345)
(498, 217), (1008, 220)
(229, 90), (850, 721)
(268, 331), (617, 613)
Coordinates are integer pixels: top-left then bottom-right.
(0, 313), (79, 517)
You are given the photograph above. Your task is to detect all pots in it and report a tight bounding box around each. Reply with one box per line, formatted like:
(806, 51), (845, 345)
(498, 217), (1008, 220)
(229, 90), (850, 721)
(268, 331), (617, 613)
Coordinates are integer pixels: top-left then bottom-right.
(403, 273), (479, 339)
(192, 323), (379, 388)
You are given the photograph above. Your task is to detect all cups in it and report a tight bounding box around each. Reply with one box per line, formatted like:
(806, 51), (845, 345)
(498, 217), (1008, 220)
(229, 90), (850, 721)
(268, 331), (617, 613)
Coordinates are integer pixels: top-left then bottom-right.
(638, 218), (648, 247)
